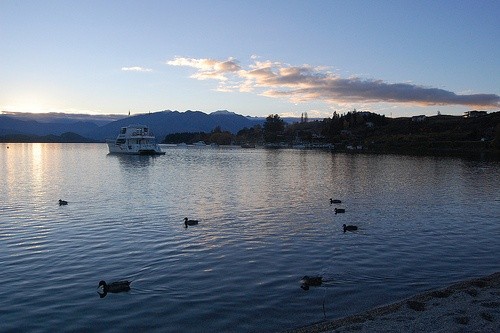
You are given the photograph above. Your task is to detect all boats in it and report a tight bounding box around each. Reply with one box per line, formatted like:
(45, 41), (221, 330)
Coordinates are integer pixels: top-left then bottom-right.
(105, 124), (166, 156)
(241, 143), (255, 148)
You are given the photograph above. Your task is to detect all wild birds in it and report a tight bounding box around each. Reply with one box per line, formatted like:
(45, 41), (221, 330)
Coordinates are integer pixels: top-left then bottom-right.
(342, 223), (358, 232)
(329, 198), (341, 203)
(58, 199), (68, 205)
(300, 275), (323, 286)
(96, 289), (130, 298)
(183, 217), (199, 226)
(334, 208), (345, 213)
(96, 280), (132, 292)
